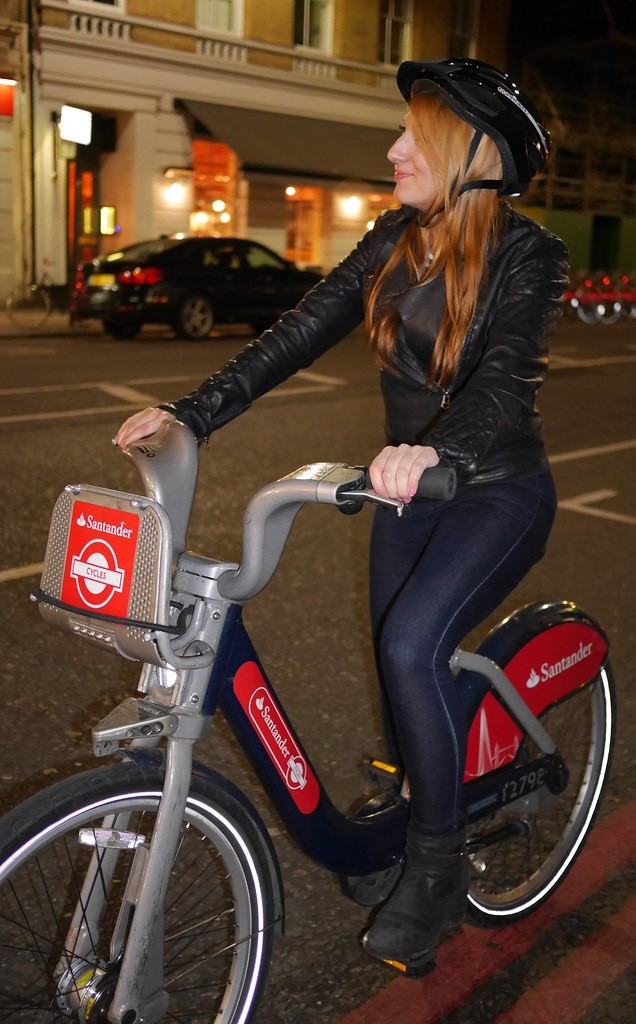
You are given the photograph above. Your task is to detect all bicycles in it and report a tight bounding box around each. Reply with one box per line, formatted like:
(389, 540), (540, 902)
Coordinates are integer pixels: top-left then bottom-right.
(566, 271), (636, 328)
(6, 257), (75, 330)
(0, 419), (616, 1024)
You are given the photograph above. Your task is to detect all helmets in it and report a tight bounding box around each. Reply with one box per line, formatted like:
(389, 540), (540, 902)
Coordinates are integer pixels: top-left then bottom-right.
(395, 56), (553, 199)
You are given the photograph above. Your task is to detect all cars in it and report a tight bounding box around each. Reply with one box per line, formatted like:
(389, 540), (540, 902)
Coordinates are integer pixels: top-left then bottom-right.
(73, 236), (326, 338)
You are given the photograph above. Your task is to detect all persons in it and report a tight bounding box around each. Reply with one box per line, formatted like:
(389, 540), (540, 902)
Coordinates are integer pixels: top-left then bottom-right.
(115, 58), (558, 961)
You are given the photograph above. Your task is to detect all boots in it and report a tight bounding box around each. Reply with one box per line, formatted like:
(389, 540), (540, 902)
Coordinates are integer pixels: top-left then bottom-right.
(352, 864), (404, 908)
(360, 811), (472, 962)
